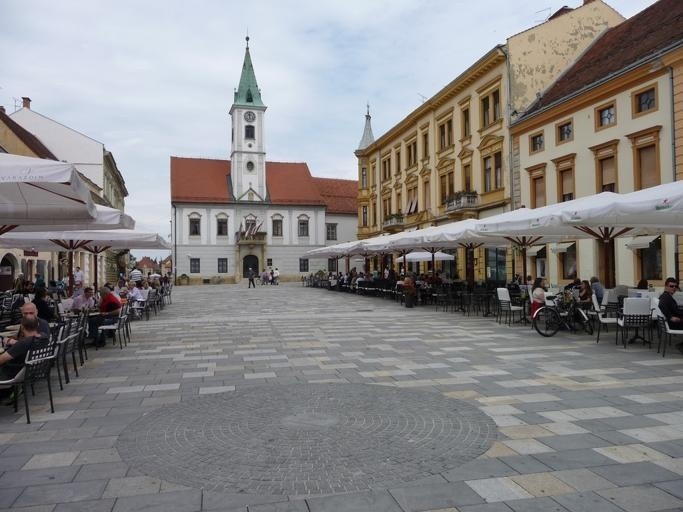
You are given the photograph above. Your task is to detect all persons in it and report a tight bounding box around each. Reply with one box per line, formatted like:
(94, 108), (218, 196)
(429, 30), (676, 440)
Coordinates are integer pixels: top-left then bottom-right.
(0, 266), (168, 406)
(260, 268), (280, 285)
(511, 272), (683, 354)
(301, 266), (453, 308)
(247, 267), (256, 288)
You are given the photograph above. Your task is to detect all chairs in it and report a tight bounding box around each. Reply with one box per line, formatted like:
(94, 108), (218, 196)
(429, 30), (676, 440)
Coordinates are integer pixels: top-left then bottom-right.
(298, 262), (535, 317)
(494, 285), (682, 358)
(0, 272), (176, 427)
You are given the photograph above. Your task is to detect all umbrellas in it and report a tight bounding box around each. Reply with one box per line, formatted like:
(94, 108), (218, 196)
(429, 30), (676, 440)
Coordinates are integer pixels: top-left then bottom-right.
(0, 152), (172, 297)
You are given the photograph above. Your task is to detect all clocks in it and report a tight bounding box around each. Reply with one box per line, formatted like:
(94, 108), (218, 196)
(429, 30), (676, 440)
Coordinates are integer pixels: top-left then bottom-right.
(243, 109), (256, 123)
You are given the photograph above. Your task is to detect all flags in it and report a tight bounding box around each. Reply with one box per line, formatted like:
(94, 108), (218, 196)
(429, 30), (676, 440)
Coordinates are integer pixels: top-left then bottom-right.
(236, 223), (262, 242)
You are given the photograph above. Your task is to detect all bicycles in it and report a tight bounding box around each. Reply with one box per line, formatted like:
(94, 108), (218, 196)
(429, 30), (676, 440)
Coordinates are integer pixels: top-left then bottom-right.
(531, 282), (595, 338)
